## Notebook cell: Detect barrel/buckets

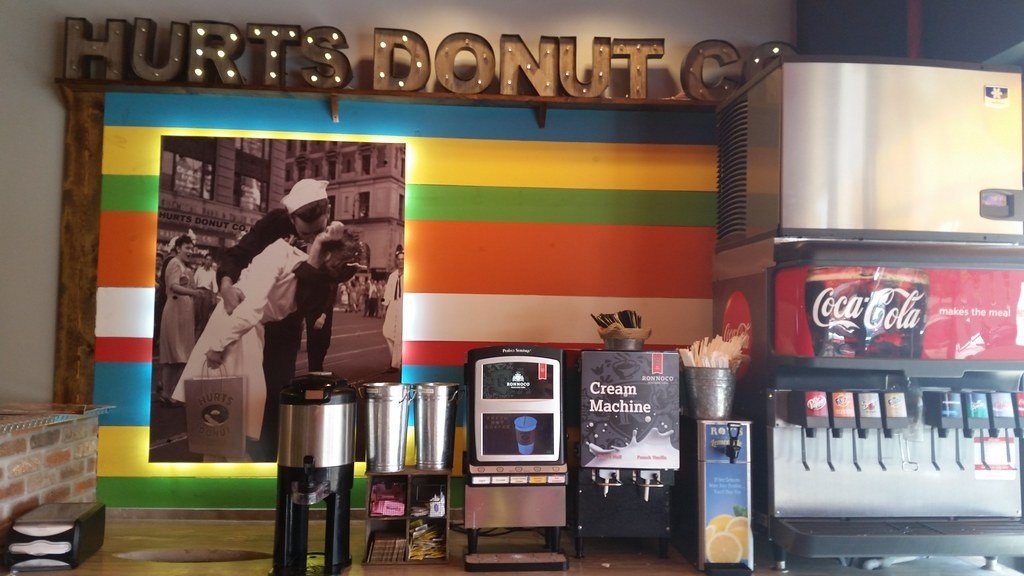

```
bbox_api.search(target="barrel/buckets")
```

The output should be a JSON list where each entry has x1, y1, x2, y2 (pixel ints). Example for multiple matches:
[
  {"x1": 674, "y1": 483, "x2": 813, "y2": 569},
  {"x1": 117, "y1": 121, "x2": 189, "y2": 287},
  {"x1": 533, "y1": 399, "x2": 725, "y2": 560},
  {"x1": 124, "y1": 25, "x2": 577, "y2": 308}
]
[
  {"x1": 680, "y1": 364, "x2": 740, "y2": 419},
  {"x1": 411, "y1": 381, "x2": 460, "y2": 471},
  {"x1": 358, "y1": 381, "x2": 417, "y2": 473}
]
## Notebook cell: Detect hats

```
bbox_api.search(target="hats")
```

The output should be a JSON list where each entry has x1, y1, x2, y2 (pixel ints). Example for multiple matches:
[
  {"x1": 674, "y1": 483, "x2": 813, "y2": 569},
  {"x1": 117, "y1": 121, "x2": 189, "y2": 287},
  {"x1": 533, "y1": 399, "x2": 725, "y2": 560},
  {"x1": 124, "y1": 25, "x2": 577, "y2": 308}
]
[{"x1": 280, "y1": 179, "x2": 329, "y2": 215}]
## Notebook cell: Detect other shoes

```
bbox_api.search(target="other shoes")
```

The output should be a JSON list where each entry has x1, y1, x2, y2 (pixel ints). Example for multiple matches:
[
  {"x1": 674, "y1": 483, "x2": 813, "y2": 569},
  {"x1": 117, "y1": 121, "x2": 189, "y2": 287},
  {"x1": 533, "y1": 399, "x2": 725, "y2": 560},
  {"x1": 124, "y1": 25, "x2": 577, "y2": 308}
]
[
  {"x1": 386, "y1": 367, "x2": 400, "y2": 373},
  {"x1": 159, "y1": 391, "x2": 178, "y2": 405}
]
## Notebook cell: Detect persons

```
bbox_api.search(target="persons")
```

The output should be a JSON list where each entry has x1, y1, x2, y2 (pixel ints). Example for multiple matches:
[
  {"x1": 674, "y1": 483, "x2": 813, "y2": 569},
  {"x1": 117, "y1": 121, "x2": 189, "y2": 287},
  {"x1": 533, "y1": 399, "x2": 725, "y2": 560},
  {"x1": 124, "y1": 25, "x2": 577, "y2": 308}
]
[{"x1": 154, "y1": 177, "x2": 405, "y2": 462}]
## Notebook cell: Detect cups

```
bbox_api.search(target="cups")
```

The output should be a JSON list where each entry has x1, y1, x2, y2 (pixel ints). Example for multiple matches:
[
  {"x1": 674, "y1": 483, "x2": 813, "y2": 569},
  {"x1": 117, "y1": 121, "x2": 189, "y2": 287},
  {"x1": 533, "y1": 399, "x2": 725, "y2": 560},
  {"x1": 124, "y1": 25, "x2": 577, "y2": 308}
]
[
  {"x1": 514, "y1": 417, "x2": 537, "y2": 454},
  {"x1": 804, "y1": 266, "x2": 929, "y2": 359}
]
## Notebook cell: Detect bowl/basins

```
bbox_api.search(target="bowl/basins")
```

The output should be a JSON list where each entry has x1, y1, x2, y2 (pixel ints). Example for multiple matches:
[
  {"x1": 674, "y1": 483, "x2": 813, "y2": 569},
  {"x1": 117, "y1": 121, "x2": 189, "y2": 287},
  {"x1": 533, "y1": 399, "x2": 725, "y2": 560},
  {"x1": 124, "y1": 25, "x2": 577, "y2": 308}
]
[{"x1": 596, "y1": 328, "x2": 652, "y2": 350}]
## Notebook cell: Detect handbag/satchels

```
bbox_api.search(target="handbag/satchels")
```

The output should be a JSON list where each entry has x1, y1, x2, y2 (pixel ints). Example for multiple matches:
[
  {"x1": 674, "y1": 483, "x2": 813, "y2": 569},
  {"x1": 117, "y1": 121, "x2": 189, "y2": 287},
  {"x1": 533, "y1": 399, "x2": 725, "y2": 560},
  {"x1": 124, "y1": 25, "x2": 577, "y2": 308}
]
[{"x1": 185, "y1": 359, "x2": 246, "y2": 457}]
